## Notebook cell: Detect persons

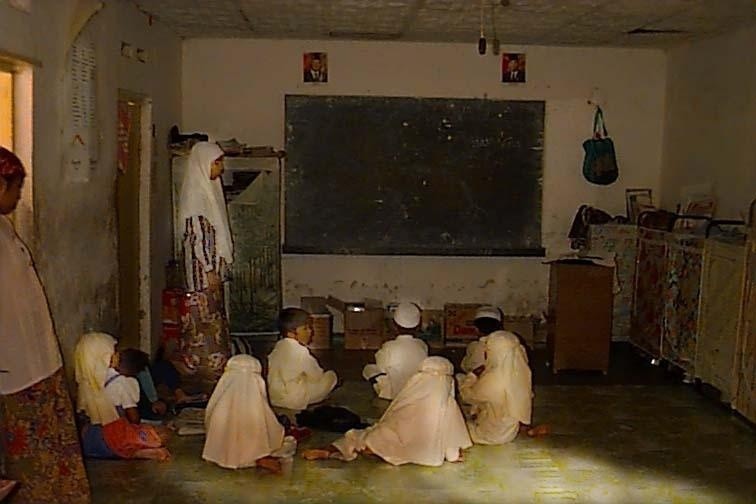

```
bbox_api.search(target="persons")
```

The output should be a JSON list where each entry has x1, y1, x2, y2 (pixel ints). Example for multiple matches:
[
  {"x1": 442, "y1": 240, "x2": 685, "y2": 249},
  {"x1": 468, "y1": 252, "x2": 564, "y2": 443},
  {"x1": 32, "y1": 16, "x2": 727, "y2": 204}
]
[
  {"x1": 502, "y1": 54, "x2": 525, "y2": 82},
  {"x1": 201, "y1": 352, "x2": 300, "y2": 475},
  {"x1": 72, "y1": 330, "x2": 171, "y2": 459},
  {"x1": 267, "y1": 305, "x2": 344, "y2": 413},
  {"x1": 0, "y1": 146, "x2": 95, "y2": 503},
  {"x1": 361, "y1": 302, "x2": 432, "y2": 403},
  {"x1": 304, "y1": 53, "x2": 328, "y2": 82},
  {"x1": 302, "y1": 355, "x2": 475, "y2": 468},
  {"x1": 172, "y1": 141, "x2": 238, "y2": 396},
  {"x1": 116, "y1": 344, "x2": 208, "y2": 423},
  {"x1": 458, "y1": 305, "x2": 503, "y2": 372},
  {"x1": 460, "y1": 329, "x2": 553, "y2": 446}
]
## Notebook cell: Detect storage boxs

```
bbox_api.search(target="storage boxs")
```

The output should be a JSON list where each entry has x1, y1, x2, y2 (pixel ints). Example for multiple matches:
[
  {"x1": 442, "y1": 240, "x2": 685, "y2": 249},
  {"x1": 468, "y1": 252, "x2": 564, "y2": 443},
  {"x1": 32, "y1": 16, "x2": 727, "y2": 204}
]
[{"x1": 299, "y1": 294, "x2": 542, "y2": 350}]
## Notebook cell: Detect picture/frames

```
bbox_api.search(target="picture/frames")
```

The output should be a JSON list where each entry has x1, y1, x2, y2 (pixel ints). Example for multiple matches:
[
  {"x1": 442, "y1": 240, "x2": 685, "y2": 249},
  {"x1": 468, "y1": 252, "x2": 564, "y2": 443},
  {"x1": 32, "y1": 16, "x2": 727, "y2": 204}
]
[
  {"x1": 300, "y1": 49, "x2": 330, "y2": 85},
  {"x1": 499, "y1": 50, "x2": 528, "y2": 85}
]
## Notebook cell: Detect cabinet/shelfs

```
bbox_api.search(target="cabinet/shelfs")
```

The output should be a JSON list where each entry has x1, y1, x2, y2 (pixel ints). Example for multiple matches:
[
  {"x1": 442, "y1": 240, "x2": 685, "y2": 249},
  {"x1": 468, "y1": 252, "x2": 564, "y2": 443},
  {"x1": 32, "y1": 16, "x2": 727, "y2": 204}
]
[{"x1": 169, "y1": 155, "x2": 282, "y2": 340}]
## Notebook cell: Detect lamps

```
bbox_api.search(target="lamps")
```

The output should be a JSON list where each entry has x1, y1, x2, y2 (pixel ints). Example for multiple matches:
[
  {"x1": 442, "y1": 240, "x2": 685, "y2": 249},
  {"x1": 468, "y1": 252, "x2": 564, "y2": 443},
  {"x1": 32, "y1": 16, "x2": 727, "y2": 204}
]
[{"x1": 479, "y1": 5, "x2": 500, "y2": 55}]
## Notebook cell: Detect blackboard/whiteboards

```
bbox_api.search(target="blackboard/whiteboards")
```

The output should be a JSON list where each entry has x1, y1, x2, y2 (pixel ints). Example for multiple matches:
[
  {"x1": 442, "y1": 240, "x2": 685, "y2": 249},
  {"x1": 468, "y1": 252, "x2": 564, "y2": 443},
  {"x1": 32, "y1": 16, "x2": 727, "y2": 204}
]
[{"x1": 281, "y1": 92, "x2": 545, "y2": 257}]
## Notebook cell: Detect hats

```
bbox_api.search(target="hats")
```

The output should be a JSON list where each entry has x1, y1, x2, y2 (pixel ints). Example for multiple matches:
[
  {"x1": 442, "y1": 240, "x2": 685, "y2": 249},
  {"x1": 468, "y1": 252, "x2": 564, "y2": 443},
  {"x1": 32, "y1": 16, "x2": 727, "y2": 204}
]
[
  {"x1": 393, "y1": 303, "x2": 421, "y2": 328},
  {"x1": 1, "y1": 146, "x2": 26, "y2": 174},
  {"x1": 474, "y1": 305, "x2": 501, "y2": 320}
]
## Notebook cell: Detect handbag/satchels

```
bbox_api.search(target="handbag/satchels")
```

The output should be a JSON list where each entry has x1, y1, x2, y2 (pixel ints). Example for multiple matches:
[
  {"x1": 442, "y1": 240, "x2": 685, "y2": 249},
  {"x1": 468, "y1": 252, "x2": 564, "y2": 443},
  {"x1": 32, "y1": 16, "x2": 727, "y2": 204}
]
[
  {"x1": 583, "y1": 136, "x2": 618, "y2": 184},
  {"x1": 568, "y1": 205, "x2": 627, "y2": 248}
]
